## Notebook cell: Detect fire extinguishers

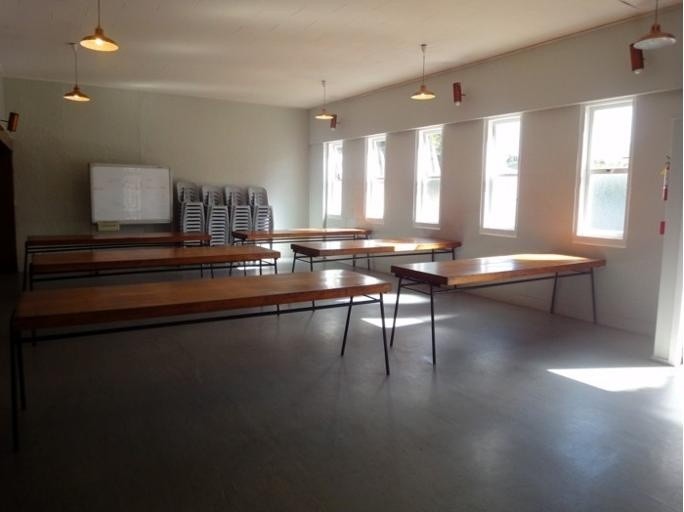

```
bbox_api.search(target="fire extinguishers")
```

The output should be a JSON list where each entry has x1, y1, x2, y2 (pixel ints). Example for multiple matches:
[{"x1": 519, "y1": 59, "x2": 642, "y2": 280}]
[{"x1": 662, "y1": 154, "x2": 671, "y2": 200}]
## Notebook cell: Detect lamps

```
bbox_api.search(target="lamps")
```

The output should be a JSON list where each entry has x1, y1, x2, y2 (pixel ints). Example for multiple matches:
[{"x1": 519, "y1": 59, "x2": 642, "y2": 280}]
[
  {"x1": 631, "y1": 0, "x2": 676, "y2": 50},
  {"x1": 453, "y1": 82, "x2": 466, "y2": 109},
  {"x1": 81, "y1": 0, "x2": 120, "y2": 52},
  {"x1": 1, "y1": 112, "x2": 19, "y2": 132},
  {"x1": 62, "y1": 43, "x2": 92, "y2": 103},
  {"x1": 408, "y1": 44, "x2": 436, "y2": 100},
  {"x1": 330, "y1": 114, "x2": 339, "y2": 130},
  {"x1": 629, "y1": 43, "x2": 644, "y2": 74},
  {"x1": 313, "y1": 79, "x2": 334, "y2": 119}
]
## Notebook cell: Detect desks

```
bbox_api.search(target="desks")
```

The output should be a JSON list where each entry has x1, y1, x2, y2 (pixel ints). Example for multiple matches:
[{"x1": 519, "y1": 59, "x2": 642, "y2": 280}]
[
  {"x1": 389, "y1": 252, "x2": 606, "y2": 365},
  {"x1": 229, "y1": 227, "x2": 371, "y2": 278},
  {"x1": 28, "y1": 246, "x2": 281, "y2": 349},
  {"x1": 21, "y1": 231, "x2": 216, "y2": 291},
  {"x1": 291, "y1": 236, "x2": 463, "y2": 311},
  {"x1": 8, "y1": 270, "x2": 389, "y2": 453}
]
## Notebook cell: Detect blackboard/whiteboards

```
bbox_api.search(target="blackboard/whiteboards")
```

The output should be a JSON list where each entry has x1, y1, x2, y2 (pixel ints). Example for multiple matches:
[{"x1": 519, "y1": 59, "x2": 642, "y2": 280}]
[{"x1": 90, "y1": 163, "x2": 173, "y2": 224}]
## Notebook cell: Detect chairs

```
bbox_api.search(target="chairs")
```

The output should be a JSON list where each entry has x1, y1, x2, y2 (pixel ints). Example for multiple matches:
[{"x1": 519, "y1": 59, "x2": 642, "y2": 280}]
[{"x1": 176, "y1": 181, "x2": 270, "y2": 264}]
